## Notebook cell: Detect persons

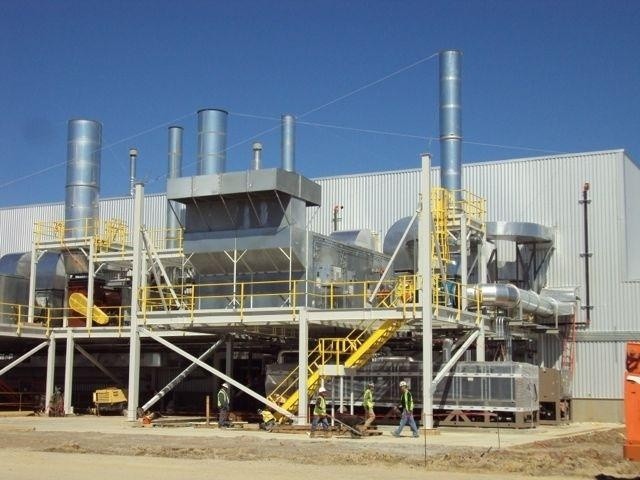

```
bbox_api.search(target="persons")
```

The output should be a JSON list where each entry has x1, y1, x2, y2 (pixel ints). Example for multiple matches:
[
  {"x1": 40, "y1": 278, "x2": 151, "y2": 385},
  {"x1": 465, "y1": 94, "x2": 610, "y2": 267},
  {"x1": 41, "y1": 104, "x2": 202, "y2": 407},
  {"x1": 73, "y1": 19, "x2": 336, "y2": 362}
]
[
  {"x1": 217, "y1": 382, "x2": 230, "y2": 425},
  {"x1": 309, "y1": 386, "x2": 331, "y2": 438},
  {"x1": 257, "y1": 409, "x2": 276, "y2": 432},
  {"x1": 362, "y1": 384, "x2": 375, "y2": 431},
  {"x1": 391, "y1": 381, "x2": 419, "y2": 437}
]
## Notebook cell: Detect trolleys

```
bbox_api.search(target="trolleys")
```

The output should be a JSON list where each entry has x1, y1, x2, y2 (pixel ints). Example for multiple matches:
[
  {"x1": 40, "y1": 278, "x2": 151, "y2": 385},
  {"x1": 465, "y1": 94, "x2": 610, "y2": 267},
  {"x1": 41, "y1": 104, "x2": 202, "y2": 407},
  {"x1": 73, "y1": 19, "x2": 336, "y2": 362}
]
[
  {"x1": 322, "y1": 411, "x2": 365, "y2": 439},
  {"x1": 222, "y1": 419, "x2": 249, "y2": 429}
]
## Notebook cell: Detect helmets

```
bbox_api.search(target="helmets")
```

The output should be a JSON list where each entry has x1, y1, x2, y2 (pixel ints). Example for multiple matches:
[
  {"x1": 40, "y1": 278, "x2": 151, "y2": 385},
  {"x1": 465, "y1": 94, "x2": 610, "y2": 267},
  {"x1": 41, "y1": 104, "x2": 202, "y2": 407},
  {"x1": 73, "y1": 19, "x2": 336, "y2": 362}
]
[
  {"x1": 222, "y1": 383, "x2": 229, "y2": 389},
  {"x1": 399, "y1": 380, "x2": 408, "y2": 387},
  {"x1": 319, "y1": 387, "x2": 327, "y2": 393}
]
